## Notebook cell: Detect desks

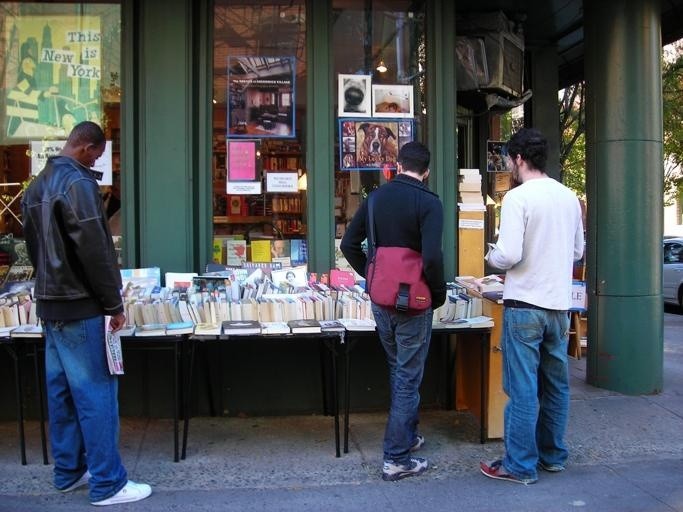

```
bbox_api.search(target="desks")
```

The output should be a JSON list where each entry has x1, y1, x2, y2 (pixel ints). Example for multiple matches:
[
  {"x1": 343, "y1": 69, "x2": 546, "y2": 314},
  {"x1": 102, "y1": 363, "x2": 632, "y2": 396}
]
[
  {"x1": 1, "y1": 315, "x2": 28, "y2": 464},
  {"x1": 28, "y1": 316, "x2": 189, "y2": 466},
  {"x1": 181, "y1": 315, "x2": 346, "y2": 460},
  {"x1": 455, "y1": 276, "x2": 510, "y2": 438},
  {"x1": 342, "y1": 315, "x2": 495, "y2": 454}
]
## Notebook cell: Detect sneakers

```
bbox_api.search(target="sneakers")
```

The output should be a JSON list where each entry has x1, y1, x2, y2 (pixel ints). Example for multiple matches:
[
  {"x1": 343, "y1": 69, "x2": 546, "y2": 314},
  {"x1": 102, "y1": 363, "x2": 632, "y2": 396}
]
[
  {"x1": 91, "y1": 479, "x2": 151, "y2": 506},
  {"x1": 62, "y1": 471, "x2": 92, "y2": 493},
  {"x1": 382, "y1": 434, "x2": 428, "y2": 481},
  {"x1": 480, "y1": 456, "x2": 565, "y2": 484}
]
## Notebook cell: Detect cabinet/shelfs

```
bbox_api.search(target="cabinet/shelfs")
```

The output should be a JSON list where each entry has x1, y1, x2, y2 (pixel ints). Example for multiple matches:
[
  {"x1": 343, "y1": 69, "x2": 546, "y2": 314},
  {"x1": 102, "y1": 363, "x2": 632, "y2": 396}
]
[
  {"x1": 100, "y1": 99, "x2": 345, "y2": 245},
  {"x1": 568, "y1": 246, "x2": 588, "y2": 359},
  {"x1": 459, "y1": 210, "x2": 486, "y2": 278}
]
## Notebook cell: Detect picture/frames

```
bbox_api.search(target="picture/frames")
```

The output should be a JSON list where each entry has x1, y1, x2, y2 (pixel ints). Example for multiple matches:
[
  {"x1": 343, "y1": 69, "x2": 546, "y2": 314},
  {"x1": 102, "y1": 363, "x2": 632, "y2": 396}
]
[
  {"x1": 225, "y1": 54, "x2": 297, "y2": 140},
  {"x1": 372, "y1": 83, "x2": 415, "y2": 117},
  {"x1": 337, "y1": 72, "x2": 373, "y2": 119},
  {"x1": 338, "y1": 116, "x2": 416, "y2": 171}
]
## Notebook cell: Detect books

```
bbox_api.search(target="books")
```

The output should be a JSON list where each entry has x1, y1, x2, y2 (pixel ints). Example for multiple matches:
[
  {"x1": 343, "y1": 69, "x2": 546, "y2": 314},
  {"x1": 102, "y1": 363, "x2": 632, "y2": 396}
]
[
  {"x1": 457, "y1": 168, "x2": 487, "y2": 212},
  {"x1": 431, "y1": 273, "x2": 506, "y2": 328},
  {"x1": 224, "y1": 264, "x2": 377, "y2": 334},
  {"x1": 118, "y1": 268, "x2": 244, "y2": 336},
  {"x1": 483, "y1": 243, "x2": 496, "y2": 262},
  {"x1": 212, "y1": 129, "x2": 308, "y2": 267},
  {"x1": 1, "y1": 265, "x2": 44, "y2": 338}
]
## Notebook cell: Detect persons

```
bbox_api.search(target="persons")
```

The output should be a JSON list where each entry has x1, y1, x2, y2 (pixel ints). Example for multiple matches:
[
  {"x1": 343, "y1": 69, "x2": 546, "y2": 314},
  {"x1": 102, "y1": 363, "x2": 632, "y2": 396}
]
[
  {"x1": 340, "y1": 140, "x2": 447, "y2": 481},
  {"x1": 479, "y1": 128, "x2": 584, "y2": 485},
  {"x1": 21, "y1": 121, "x2": 153, "y2": 506}
]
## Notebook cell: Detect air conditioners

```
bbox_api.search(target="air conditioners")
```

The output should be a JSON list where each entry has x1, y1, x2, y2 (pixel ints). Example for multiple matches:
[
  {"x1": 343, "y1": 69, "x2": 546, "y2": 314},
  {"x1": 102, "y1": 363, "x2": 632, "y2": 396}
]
[{"x1": 471, "y1": 28, "x2": 526, "y2": 99}]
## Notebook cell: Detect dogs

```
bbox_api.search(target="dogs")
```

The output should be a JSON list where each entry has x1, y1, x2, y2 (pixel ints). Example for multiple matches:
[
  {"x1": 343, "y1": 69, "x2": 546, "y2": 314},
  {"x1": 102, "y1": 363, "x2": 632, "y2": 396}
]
[{"x1": 356, "y1": 123, "x2": 398, "y2": 165}]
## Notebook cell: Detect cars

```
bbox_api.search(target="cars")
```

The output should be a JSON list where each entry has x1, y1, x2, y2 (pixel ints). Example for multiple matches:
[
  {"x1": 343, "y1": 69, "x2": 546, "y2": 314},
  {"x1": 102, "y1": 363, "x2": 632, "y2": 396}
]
[{"x1": 663, "y1": 239, "x2": 683, "y2": 310}]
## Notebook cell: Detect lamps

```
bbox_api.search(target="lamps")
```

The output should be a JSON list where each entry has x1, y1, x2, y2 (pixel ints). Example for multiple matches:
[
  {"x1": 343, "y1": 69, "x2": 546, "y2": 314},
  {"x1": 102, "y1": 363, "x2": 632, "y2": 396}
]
[{"x1": 375, "y1": 55, "x2": 388, "y2": 73}]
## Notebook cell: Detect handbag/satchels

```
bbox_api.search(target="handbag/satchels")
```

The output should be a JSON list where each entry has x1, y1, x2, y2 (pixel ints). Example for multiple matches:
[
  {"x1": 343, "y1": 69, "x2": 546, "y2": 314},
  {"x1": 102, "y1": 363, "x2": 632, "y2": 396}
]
[{"x1": 367, "y1": 244, "x2": 433, "y2": 315}]
